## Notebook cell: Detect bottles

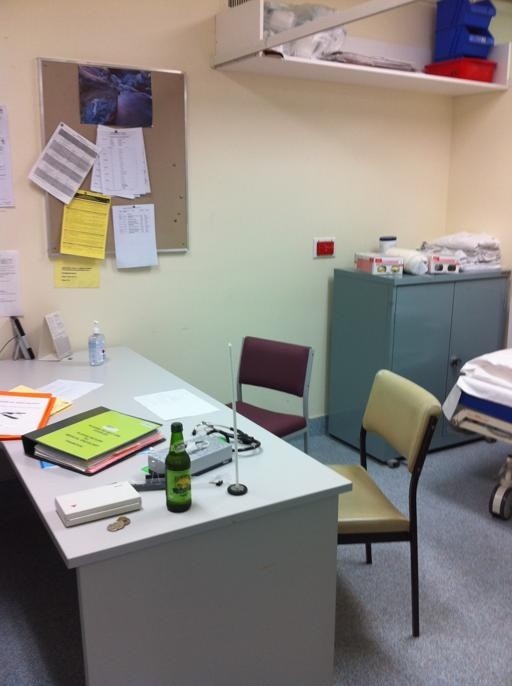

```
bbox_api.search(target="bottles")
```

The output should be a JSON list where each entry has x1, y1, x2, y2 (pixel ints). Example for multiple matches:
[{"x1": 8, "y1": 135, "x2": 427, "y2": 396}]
[
  {"x1": 378, "y1": 235, "x2": 398, "y2": 252},
  {"x1": 86, "y1": 319, "x2": 107, "y2": 367},
  {"x1": 165, "y1": 420, "x2": 192, "y2": 513}
]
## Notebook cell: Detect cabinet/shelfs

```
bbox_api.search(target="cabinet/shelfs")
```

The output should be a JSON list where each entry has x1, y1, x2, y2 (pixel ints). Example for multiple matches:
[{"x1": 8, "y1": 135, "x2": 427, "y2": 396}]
[{"x1": 325, "y1": 266, "x2": 512, "y2": 468}]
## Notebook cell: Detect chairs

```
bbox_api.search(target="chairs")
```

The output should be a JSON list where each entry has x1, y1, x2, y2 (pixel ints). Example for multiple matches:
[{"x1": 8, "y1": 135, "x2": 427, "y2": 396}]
[
  {"x1": 324, "y1": 370, "x2": 442, "y2": 638},
  {"x1": 226, "y1": 336, "x2": 315, "y2": 456}
]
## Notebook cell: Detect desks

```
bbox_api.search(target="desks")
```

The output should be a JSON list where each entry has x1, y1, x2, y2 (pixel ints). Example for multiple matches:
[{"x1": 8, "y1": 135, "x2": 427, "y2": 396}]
[{"x1": 1, "y1": 343, "x2": 354, "y2": 686}]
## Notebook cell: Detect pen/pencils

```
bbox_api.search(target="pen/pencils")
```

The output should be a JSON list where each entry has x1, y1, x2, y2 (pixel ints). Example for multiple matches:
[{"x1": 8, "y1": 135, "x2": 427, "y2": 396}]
[{"x1": 1, "y1": 412, "x2": 26, "y2": 419}]
